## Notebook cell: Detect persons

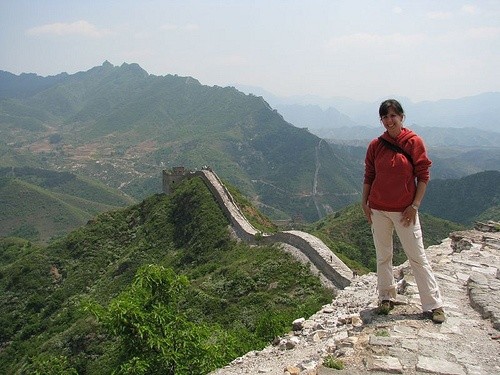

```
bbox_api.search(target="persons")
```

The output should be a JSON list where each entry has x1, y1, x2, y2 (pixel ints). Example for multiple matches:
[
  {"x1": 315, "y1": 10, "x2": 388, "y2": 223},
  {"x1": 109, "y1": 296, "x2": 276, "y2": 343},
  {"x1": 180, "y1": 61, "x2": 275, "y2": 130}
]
[{"x1": 362, "y1": 99, "x2": 445, "y2": 323}]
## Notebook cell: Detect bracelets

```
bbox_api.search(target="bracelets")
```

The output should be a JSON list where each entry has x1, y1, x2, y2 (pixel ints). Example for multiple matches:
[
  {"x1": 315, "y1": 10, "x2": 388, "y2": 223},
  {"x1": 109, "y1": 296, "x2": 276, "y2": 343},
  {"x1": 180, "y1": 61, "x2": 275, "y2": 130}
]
[{"x1": 412, "y1": 204, "x2": 419, "y2": 209}]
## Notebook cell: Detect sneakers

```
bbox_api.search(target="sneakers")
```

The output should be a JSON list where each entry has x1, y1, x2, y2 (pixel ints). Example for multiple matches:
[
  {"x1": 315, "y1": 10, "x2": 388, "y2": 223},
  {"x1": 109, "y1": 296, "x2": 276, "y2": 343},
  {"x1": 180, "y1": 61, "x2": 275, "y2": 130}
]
[
  {"x1": 378, "y1": 300, "x2": 394, "y2": 315},
  {"x1": 432, "y1": 307, "x2": 445, "y2": 322}
]
